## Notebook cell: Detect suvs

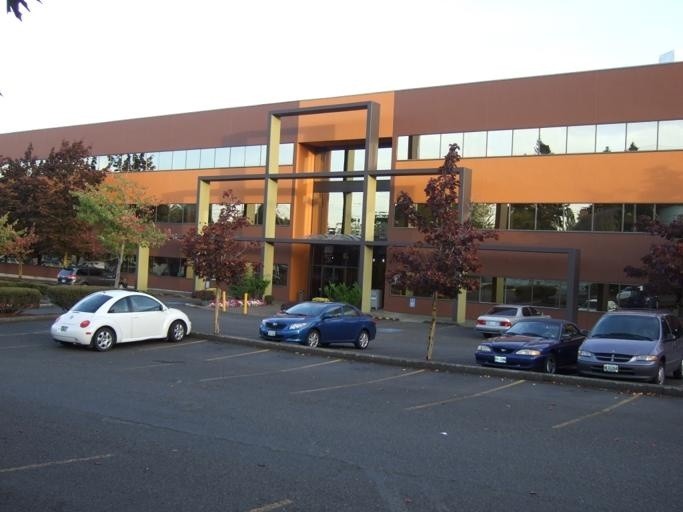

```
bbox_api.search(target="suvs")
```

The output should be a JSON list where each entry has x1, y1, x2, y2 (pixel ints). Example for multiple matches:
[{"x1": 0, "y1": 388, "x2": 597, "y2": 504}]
[{"x1": 58, "y1": 261, "x2": 128, "y2": 291}]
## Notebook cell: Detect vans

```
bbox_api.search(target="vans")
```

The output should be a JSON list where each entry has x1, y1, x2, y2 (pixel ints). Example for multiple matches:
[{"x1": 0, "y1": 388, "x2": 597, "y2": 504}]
[{"x1": 577, "y1": 307, "x2": 682, "y2": 385}]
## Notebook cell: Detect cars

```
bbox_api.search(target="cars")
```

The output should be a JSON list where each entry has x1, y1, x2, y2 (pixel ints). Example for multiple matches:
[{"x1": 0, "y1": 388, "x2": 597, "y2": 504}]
[
  {"x1": 49, "y1": 289, "x2": 193, "y2": 351},
  {"x1": 477, "y1": 304, "x2": 553, "y2": 342},
  {"x1": 477, "y1": 318, "x2": 588, "y2": 377},
  {"x1": 258, "y1": 298, "x2": 377, "y2": 352},
  {"x1": 579, "y1": 286, "x2": 660, "y2": 312}
]
